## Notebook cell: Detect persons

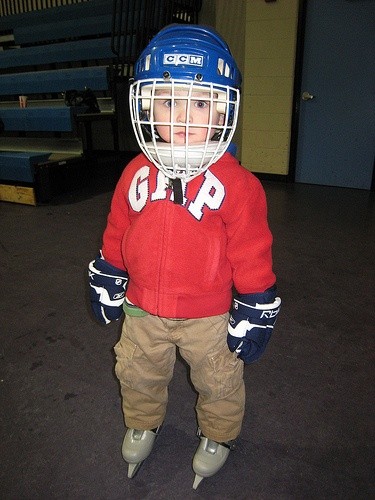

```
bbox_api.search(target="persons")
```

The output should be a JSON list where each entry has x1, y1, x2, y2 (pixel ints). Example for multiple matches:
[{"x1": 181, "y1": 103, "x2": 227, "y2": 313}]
[{"x1": 89, "y1": 24, "x2": 280, "y2": 478}]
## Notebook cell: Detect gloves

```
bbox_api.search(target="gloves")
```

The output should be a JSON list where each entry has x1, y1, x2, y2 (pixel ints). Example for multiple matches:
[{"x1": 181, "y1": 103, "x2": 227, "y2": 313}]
[
  {"x1": 227, "y1": 296, "x2": 281, "y2": 365},
  {"x1": 87, "y1": 260, "x2": 129, "y2": 326}
]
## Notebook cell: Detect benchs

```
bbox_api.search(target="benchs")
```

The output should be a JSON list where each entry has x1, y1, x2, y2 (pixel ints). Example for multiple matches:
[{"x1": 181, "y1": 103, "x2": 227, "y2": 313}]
[{"x1": 0, "y1": 0, "x2": 202, "y2": 208}]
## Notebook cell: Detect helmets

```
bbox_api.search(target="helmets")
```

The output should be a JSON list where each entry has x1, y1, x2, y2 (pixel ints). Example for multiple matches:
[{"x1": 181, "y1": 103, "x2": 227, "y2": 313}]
[{"x1": 129, "y1": 21, "x2": 240, "y2": 167}]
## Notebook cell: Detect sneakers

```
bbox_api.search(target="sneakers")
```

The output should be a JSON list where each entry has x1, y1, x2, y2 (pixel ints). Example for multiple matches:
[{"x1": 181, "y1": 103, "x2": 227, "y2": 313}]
[
  {"x1": 192, "y1": 427, "x2": 237, "y2": 490},
  {"x1": 122, "y1": 426, "x2": 160, "y2": 478}
]
